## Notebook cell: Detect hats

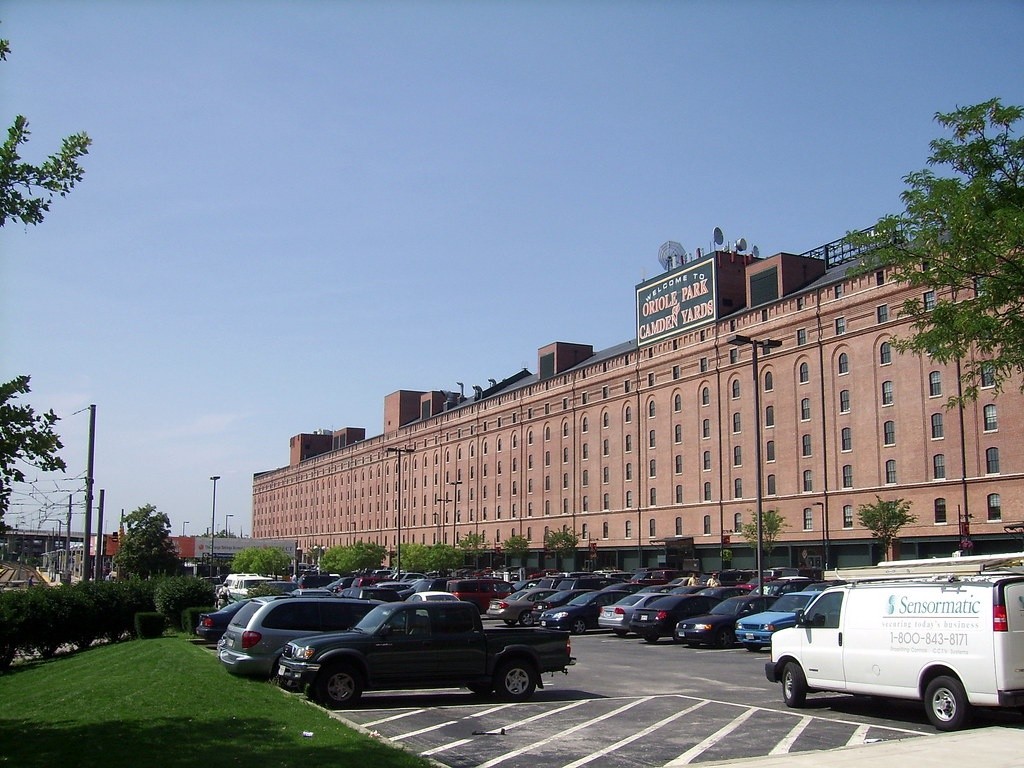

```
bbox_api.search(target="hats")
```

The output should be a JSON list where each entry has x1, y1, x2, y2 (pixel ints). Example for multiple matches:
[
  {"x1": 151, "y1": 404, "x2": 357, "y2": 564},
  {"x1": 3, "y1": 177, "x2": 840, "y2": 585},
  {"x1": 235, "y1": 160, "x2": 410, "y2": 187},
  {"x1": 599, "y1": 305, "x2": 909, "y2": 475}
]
[
  {"x1": 223, "y1": 582, "x2": 228, "y2": 585},
  {"x1": 712, "y1": 572, "x2": 717, "y2": 576}
]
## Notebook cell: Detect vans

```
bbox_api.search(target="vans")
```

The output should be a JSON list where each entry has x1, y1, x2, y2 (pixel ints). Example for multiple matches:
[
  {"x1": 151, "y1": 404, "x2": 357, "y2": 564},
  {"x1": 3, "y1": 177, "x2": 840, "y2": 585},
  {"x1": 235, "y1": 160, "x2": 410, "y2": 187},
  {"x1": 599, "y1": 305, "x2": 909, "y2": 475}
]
[{"x1": 762, "y1": 570, "x2": 1023, "y2": 732}]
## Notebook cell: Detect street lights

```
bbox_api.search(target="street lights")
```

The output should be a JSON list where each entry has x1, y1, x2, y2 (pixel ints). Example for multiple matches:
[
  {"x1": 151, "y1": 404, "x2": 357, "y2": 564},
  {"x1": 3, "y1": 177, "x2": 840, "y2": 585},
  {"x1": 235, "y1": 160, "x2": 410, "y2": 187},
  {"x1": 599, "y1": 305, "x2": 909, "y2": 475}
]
[
  {"x1": 813, "y1": 501, "x2": 826, "y2": 570},
  {"x1": 448, "y1": 481, "x2": 463, "y2": 549},
  {"x1": 385, "y1": 447, "x2": 416, "y2": 582},
  {"x1": 726, "y1": 334, "x2": 782, "y2": 597},
  {"x1": 314, "y1": 545, "x2": 326, "y2": 575},
  {"x1": 209, "y1": 475, "x2": 221, "y2": 592},
  {"x1": 225, "y1": 514, "x2": 234, "y2": 538},
  {"x1": 437, "y1": 498, "x2": 452, "y2": 546},
  {"x1": 182, "y1": 521, "x2": 190, "y2": 537}
]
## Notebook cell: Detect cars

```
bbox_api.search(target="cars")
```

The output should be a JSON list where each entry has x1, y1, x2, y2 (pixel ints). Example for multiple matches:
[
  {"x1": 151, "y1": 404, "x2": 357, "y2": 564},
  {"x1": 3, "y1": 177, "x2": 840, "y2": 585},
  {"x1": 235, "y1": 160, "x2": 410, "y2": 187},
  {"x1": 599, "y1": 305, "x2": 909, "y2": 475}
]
[{"x1": 192, "y1": 568, "x2": 846, "y2": 691}]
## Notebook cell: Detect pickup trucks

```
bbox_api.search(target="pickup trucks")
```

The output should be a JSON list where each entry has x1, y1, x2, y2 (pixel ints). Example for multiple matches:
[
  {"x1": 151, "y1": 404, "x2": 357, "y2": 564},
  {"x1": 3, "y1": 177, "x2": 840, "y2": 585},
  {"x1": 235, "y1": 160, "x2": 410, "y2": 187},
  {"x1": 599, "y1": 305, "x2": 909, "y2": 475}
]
[{"x1": 277, "y1": 598, "x2": 577, "y2": 710}]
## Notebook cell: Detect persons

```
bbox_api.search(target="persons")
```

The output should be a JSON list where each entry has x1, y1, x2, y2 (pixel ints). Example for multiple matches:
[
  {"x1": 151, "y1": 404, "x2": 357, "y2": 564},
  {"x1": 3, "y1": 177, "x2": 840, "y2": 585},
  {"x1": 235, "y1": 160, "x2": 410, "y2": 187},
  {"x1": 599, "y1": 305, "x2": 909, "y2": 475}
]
[
  {"x1": 292, "y1": 573, "x2": 298, "y2": 584},
  {"x1": 688, "y1": 573, "x2": 701, "y2": 586},
  {"x1": 298, "y1": 574, "x2": 306, "y2": 589},
  {"x1": 707, "y1": 573, "x2": 721, "y2": 588},
  {"x1": 28, "y1": 575, "x2": 35, "y2": 587},
  {"x1": 218, "y1": 582, "x2": 231, "y2": 611}
]
